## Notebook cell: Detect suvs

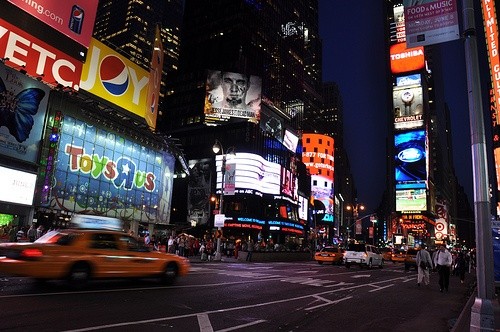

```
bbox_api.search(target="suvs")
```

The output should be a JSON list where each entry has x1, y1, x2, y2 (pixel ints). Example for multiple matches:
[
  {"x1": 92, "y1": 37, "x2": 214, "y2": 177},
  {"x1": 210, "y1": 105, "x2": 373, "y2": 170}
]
[
  {"x1": 343, "y1": 244, "x2": 384, "y2": 270},
  {"x1": 379, "y1": 247, "x2": 392, "y2": 261},
  {"x1": 403, "y1": 247, "x2": 424, "y2": 272}
]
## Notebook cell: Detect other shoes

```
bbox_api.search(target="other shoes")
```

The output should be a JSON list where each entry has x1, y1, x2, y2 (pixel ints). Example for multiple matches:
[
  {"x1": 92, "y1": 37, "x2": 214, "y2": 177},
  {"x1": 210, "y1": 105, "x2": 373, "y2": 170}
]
[
  {"x1": 440, "y1": 288, "x2": 443, "y2": 293},
  {"x1": 445, "y1": 288, "x2": 448, "y2": 292}
]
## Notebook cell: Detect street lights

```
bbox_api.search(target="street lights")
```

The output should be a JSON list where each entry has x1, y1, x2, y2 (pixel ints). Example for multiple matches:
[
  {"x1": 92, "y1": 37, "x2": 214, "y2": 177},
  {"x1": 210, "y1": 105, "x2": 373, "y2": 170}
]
[
  {"x1": 347, "y1": 202, "x2": 364, "y2": 239},
  {"x1": 213, "y1": 138, "x2": 225, "y2": 262}
]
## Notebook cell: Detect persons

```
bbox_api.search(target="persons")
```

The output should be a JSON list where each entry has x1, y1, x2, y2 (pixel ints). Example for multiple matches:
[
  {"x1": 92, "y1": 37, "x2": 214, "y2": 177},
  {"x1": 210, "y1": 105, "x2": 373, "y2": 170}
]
[
  {"x1": 401, "y1": 243, "x2": 477, "y2": 293},
  {"x1": 211, "y1": 71, "x2": 253, "y2": 111},
  {"x1": 130, "y1": 231, "x2": 325, "y2": 262},
  {"x1": 0, "y1": 223, "x2": 58, "y2": 243}
]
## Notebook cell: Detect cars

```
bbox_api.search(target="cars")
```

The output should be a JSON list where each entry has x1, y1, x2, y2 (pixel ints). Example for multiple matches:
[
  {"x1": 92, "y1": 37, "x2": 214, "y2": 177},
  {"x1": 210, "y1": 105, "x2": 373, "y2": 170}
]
[
  {"x1": 314, "y1": 245, "x2": 345, "y2": 264},
  {"x1": 0, "y1": 216, "x2": 191, "y2": 286},
  {"x1": 391, "y1": 250, "x2": 407, "y2": 264}
]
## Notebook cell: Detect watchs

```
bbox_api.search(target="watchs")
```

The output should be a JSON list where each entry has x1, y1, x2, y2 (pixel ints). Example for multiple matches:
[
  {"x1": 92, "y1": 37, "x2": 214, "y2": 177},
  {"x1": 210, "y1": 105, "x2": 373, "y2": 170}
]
[{"x1": 402, "y1": 87, "x2": 414, "y2": 118}]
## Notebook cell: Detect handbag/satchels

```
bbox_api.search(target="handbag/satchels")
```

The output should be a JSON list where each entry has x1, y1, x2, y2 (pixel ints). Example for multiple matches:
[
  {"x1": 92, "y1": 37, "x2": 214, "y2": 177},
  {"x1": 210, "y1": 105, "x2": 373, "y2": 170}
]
[{"x1": 420, "y1": 260, "x2": 428, "y2": 269}]
